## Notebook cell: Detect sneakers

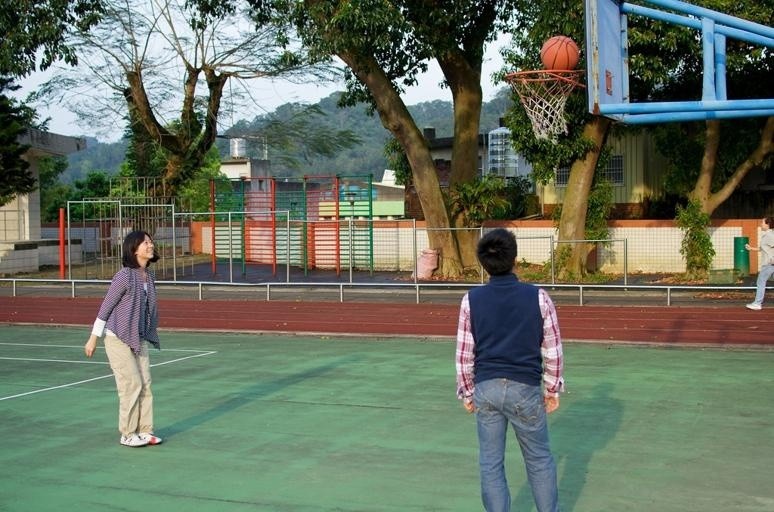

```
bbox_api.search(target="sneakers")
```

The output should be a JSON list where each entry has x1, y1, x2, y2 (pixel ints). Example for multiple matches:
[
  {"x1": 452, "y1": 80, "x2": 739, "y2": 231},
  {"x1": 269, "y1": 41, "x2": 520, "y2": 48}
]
[
  {"x1": 120, "y1": 432, "x2": 149, "y2": 446},
  {"x1": 746, "y1": 301, "x2": 762, "y2": 311},
  {"x1": 137, "y1": 432, "x2": 162, "y2": 444}
]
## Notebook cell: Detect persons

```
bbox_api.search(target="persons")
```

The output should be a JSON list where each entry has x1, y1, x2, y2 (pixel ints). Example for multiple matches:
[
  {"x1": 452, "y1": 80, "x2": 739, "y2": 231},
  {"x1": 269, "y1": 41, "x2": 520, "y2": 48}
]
[
  {"x1": 452, "y1": 227, "x2": 565, "y2": 512},
  {"x1": 744, "y1": 214, "x2": 774, "y2": 312},
  {"x1": 83, "y1": 230, "x2": 164, "y2": 448}
]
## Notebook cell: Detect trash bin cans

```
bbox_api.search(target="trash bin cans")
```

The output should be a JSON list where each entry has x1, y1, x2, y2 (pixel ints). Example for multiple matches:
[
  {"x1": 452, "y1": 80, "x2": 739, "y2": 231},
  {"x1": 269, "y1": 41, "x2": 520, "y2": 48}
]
[{"x1": 734, "y1": 237, "x2": 749, "y2": 277}]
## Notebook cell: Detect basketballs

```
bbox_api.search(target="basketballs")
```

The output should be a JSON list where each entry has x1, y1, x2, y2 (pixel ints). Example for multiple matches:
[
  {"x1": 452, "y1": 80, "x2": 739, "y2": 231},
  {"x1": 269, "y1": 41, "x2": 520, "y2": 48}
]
[{"x1": 541, "y1": 36, "x2": 579, "y2": 72}]
